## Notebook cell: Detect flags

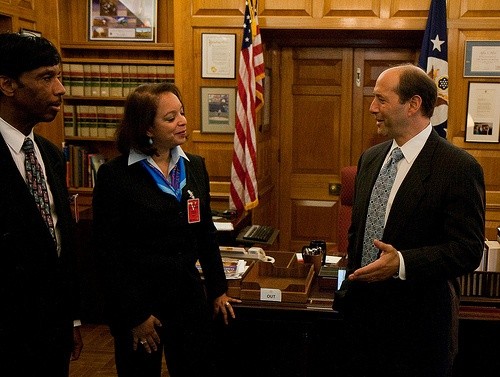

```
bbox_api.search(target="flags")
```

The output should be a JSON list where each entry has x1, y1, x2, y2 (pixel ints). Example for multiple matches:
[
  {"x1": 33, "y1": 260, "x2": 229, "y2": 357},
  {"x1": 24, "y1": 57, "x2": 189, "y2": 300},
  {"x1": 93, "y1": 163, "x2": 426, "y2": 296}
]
[
  {"x1": 417, "y1": 0, "x2": 450, "y2": 140},
  {"x1": 228, "y1": 1, "x2": 266, "y2": 228}
]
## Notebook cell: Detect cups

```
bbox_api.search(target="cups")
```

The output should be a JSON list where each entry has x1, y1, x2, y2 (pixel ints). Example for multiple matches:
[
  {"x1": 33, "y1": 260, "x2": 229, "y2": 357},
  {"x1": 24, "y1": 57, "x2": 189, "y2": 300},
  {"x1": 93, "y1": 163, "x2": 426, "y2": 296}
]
[
  {"x1": 301, "y1": 240, "x2": 327, "y2": 266},
  {"x1": 303, "y1": 252, "x2": 322, "y2": 278}
]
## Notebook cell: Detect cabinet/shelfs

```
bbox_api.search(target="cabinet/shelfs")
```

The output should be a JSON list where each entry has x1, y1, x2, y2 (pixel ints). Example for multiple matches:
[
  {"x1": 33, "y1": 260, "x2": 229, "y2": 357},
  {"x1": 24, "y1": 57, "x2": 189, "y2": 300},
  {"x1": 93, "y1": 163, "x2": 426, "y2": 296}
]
[
  {"x1": 219, "y1": 251, "x2": 316, "y2": 302},
  {"x1": 61, "y1": 57, "x2": 175, "y2": 219}
]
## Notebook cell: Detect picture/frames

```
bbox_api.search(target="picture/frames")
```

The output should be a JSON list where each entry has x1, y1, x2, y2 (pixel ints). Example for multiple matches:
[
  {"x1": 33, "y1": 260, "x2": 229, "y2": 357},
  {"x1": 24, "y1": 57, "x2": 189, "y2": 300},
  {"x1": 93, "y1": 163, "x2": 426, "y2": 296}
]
[
  {"x1": 85, "y1": 0, "x2": 158, "y2": 43},
  {"x1": 199, "y1": 85, "x2": 238, "y2": 135},
  {"x1": 20, "y1": 27, "x2": 42, "y2": 37}
]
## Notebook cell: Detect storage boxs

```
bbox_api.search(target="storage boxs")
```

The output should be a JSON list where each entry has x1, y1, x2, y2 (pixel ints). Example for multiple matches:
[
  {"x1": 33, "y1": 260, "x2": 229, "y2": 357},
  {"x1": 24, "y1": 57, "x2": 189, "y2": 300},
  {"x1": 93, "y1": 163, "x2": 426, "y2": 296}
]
[
  {"x1": 205, "y1": 254, "x2": 500, "y2": 377},
  {"x1": 456, "y1": 239, "x2": 500, "y2": 307}
]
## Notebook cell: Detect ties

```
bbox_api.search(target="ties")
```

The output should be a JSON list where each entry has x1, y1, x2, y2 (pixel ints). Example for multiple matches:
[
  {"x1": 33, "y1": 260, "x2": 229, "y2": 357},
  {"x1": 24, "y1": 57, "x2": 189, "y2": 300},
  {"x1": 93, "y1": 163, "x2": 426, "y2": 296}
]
[
  {"x1": 20, "y1": 137, "x2": 58, "y2": 253},
  {"x1": 360, "y1": 148, "x2": 405, "y2": 267}
]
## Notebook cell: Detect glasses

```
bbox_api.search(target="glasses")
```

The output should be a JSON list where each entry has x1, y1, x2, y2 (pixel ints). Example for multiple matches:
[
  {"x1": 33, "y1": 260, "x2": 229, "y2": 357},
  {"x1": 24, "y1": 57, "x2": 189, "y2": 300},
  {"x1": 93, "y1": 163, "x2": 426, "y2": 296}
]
[{"x1": 18, "y1": 29, "x2": 43, "y2": 47}]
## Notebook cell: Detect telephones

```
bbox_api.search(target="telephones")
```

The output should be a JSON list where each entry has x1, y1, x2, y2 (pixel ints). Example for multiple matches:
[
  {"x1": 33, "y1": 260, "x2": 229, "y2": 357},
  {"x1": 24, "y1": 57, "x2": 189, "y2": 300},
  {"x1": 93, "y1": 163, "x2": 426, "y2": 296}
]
[{"x1": 219, "y1": 245, "x2": 268, "y2": 262}]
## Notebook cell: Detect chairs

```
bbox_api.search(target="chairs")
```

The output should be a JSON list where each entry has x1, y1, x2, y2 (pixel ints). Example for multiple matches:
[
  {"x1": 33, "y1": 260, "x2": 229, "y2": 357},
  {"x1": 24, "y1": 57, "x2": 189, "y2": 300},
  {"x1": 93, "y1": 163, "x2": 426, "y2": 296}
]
[{"x1": 327, "y1": 165, "x2": 358, "y2": 257}]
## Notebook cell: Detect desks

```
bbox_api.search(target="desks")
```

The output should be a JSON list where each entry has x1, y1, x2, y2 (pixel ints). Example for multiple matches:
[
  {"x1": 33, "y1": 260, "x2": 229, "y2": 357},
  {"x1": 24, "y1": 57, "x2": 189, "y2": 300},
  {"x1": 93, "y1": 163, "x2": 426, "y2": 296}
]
[{"x1": 212, "y1": 207, "x2": 280, "y2": 249}]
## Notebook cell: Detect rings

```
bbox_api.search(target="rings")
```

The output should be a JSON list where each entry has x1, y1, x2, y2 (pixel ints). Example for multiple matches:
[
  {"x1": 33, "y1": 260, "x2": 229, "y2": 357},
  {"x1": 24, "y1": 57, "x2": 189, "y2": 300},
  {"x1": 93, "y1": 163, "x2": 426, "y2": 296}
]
[
  {"x1": 225, "y1": 302, "x2": 229, "y2": 306},
  {"x1": 140, "y1": 341, "x2": 147, "y2": 344}
]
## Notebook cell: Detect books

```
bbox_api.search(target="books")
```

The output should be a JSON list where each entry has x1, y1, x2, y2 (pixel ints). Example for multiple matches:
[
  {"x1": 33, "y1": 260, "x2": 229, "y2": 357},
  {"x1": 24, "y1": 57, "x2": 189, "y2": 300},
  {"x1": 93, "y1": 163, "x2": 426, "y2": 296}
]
[
  {"x1": 61, "y1": 141, "x2": 107, "y2": 190},
  {"x1": 60, "y1": 63, "x2": 176, "y2": 99},
  {"x1": 456, "y1": 227, "x2": 500, "y2": 297},
  {"x1": 62, "y1": 105, "x2": 126, "y2": 139}
]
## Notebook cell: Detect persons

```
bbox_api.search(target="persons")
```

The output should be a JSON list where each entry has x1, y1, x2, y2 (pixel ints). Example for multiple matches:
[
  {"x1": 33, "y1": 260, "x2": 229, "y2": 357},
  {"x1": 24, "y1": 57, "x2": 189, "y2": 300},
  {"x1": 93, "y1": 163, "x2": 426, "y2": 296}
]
[
  {"x1": 0, "y1": 31, "x2": 84, "y2": 377},
  {"x1": 332, "y1": 64, "x2": 487, "y2": 377},
  {"x1": 91, "y1": 83, "x2": 244, "y2": 377}
]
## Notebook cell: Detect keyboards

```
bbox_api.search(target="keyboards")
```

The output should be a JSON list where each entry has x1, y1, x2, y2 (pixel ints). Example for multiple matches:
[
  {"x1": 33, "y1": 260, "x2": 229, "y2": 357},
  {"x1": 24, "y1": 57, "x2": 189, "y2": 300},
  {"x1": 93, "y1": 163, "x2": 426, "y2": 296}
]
[{"x1": 242, "y1": 224, "x2": 273, "y2": 241}]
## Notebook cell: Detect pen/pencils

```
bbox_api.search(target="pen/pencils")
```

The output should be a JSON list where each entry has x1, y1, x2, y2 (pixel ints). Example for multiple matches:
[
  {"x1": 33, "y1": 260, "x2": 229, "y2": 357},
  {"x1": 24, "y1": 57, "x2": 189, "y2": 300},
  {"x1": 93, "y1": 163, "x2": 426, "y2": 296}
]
[{"x1": 305, "y1": 247, "x2": 323, "y2": 255}]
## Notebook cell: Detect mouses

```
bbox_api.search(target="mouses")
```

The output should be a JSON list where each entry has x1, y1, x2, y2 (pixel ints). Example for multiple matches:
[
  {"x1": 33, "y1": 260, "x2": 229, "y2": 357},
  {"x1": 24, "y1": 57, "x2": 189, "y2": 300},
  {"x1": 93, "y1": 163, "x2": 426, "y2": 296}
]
[{"x1": 223, "y1": 210, "x2": 237, "y2": 215}]
